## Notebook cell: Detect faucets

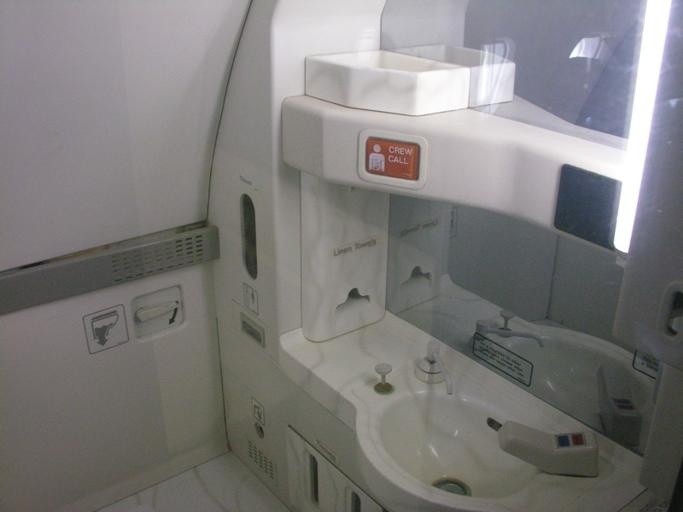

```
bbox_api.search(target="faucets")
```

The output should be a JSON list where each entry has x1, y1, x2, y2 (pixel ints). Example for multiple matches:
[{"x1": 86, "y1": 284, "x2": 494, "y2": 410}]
[
  {"x1": 476, "y1": 309, "x2": 545, "y2": 349},
  {"x1": 415, "y1": 341, "x2": 453, "y2": 394}
]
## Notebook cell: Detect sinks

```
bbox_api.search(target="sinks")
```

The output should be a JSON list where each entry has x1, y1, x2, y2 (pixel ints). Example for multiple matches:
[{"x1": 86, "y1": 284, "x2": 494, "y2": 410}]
[
  {"x1": 378, "y1": 392, "x2": 541, "y2": 500},
  {"x1": 507, "y1": 338, "x2": 654, "y2": 457}
]
